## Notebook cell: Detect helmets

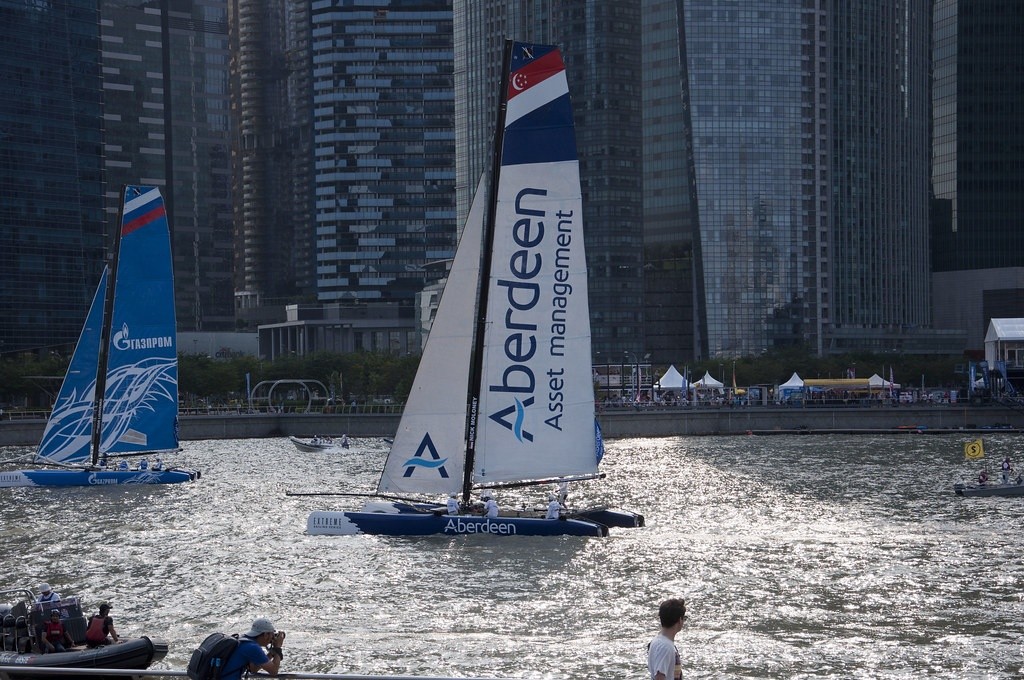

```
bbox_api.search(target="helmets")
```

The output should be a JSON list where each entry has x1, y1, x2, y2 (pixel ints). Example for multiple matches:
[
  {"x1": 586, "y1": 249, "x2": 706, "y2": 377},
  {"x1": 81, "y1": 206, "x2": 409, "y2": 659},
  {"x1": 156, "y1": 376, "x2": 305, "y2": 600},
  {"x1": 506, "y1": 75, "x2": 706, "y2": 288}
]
[
  {"x1": 548, "y1": 495, "x2": 555, "y2": 501},
  {"x1": 38, "y1": 582, "x2": 51, "y2": 592},
  {"x1": 450, "y1": 492, "x2": 456, "y2": 497},
  {"x1": 50, "y1": 609, "x2": 60, "y2": 616}
]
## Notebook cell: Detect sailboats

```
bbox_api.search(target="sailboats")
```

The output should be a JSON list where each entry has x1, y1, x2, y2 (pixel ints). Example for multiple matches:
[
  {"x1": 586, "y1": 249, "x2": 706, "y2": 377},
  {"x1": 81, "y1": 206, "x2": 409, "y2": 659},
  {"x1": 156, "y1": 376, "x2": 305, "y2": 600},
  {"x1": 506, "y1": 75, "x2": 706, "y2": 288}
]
[
  {"x1": 0, "y1": 183, "x2": 201, "y2": 487},
  {"x1": 307, "y1": 38, "x2": 645, "y2": 537}
]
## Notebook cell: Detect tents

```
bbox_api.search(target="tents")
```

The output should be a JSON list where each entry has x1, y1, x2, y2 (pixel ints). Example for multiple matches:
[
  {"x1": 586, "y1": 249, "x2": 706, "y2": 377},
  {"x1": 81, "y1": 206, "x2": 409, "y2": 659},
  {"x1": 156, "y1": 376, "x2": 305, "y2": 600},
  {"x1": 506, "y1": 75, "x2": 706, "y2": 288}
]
[
  {"x1": 869, "y1": 374, "x2": 900, "y2": 388},
  {"x1": 777, "y1": 373, "x2": 813, "y2": 391},
  {"x1": 651, "y1": 364, "x2": 724, "y2": 391}
]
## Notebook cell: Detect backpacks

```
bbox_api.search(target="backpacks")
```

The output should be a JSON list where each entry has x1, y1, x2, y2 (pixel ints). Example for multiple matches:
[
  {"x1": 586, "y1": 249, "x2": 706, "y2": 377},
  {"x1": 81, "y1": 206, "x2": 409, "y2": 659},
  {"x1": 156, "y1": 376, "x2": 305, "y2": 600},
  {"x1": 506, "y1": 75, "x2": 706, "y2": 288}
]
[{"x1": 187, "y1": 632, "x2": 254, "y2": 680}]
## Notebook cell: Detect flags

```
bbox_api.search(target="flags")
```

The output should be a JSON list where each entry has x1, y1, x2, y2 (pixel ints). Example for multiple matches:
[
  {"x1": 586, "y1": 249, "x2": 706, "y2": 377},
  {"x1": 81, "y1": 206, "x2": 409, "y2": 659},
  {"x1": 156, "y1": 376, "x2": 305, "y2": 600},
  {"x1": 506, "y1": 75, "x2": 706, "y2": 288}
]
[{"x1": 964, "y1": 438, "x2": 984, "y2": 459}]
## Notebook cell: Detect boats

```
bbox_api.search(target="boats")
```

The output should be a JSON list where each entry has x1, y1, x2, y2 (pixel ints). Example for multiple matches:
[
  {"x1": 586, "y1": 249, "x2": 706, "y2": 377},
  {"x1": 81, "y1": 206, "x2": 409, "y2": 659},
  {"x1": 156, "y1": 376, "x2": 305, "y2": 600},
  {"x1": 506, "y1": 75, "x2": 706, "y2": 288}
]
[
  {"x1": 384, "y1": 438, "x2": 394, "y2": 448},
  {"x1": 289, "y1": 434, "x2": 349, "y2": 453},
  {"x1": 953, "y1": 437, "x2": 1024, "y2": 497},
  {"x1": 0, "y1": 589, "x2": 169, "y2": 680}
]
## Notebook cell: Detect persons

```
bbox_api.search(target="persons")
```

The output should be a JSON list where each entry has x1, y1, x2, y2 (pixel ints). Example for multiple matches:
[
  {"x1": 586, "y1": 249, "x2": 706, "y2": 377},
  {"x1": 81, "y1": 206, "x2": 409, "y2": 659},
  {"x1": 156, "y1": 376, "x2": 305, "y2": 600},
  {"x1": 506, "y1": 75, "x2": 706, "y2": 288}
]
[
  {"x1": 187, "y1": 619, "x2": 286, "y2": 680},
  {"x1": 557, "y1": 476, "x2": 568, "y2": 509},
  {"x1": 351, "y1": 400, "x2": 356, "y2": 414},
  {"x1": 37, "y1": 583, "x2": 61, "y2": 619},
  {"x1": 100, "y1": 451, "x2": 109, "y2": 466},
  {"x1": 599, "y1": 390, "x2": 951, "y2": 409},
  {"x1": 447, "y1": 492, "x2": 460, "y2": 516},
  {"x1": 152, "y1": 457, "x2": 161, "y2": 471},
  {"x1": 545, "y1": 496, "x2": 565, "y2": 519},
  {"x1": 1002, "y1": 456, "x2": 1013, "y2": 482},
  {"x1": 85, "y1": 604, "x2": 123, "y2": 648},
  {"x1": 979, "y1": 470, "x2": 987, "y2": 488},
  {"x1": 483, "y1": 495, "x2": 498, "y2": 517},
  {"x1": 311, "y1": 435, "x2": 333, "y2": 445},
  {"x1": 139, "y1": 457, "x2": 148, "y2": 471},
  {"x1": 647, "y1": 599, "x2": 688, "y2": 680},
  {"x1": 41, "y1": 609, "x2": 78, "y2": 653}
]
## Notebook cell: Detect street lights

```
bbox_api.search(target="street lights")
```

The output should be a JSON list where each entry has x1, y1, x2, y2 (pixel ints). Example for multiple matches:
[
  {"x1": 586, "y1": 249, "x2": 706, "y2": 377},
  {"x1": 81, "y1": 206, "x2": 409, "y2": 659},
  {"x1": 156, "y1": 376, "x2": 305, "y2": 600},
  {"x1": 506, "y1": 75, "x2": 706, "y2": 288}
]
[
  {"x1": 624, "y1": 351, "x2": 654, "y2": 403},
  {"x1": 594, "y1": 352, "x2": 629, "y2": 402}
]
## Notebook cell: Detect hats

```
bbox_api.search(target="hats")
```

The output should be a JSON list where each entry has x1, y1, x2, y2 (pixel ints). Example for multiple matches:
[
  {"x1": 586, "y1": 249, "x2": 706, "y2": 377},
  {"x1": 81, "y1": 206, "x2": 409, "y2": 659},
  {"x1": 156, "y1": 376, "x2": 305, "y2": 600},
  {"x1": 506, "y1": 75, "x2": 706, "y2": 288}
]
[
  {"x1": 243, "y1": 618, "x2": 275, "y2": 637},
  {"x1": 100, "y1": 604, "x2": 114, "y2": 610}
]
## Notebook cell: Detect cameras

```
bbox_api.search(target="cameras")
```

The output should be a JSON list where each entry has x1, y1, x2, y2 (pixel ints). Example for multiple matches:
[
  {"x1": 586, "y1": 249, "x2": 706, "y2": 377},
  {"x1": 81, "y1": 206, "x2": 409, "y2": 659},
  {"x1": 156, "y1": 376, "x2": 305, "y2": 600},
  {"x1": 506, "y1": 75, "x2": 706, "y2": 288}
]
[{"x1": 272, "y1": 632, "x2": 286, "y2": 644}]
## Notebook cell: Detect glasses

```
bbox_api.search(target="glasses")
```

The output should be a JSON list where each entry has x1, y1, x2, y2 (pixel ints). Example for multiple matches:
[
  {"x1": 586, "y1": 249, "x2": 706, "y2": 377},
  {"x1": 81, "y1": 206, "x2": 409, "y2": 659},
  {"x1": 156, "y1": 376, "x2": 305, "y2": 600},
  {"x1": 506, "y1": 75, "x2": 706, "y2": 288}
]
[{"x1": 682, "y1": 616, "x2": 688, "y2": 621}]
